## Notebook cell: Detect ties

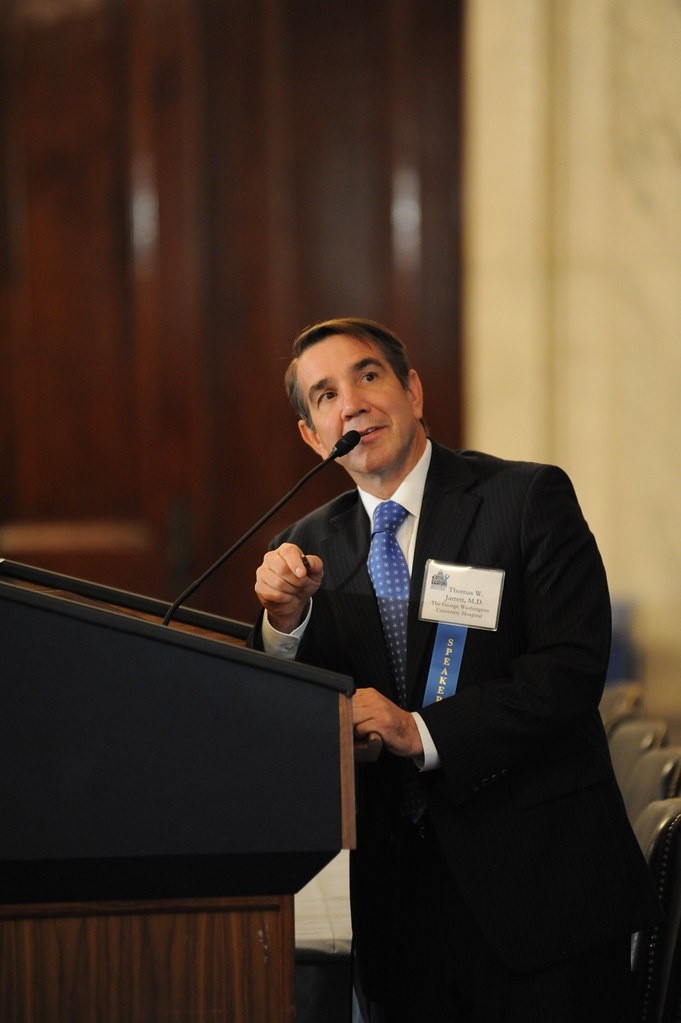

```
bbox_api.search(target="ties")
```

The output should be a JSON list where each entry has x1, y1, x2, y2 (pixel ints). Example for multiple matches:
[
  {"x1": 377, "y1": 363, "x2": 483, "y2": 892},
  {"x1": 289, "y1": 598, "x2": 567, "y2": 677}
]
[{"x1": 365, "y1": 501, "x2": 429, "y2": 825}]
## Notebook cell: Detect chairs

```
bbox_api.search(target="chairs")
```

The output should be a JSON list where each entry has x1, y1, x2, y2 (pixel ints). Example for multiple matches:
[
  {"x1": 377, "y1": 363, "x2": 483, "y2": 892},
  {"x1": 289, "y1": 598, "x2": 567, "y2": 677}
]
[{"x1": 598, "y1": 683, "x2": 681, "y2": 1023}]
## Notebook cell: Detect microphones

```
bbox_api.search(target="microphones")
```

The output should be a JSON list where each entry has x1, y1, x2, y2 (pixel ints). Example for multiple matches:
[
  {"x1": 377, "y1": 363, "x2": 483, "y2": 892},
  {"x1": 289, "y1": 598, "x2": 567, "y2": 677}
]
[{"x1": 163, "y1": 430, "x2": 361, "y2": 625}]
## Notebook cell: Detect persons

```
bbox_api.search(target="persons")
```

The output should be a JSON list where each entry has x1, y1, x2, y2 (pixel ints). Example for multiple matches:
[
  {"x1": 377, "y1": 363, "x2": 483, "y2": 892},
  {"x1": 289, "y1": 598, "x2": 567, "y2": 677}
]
[{"x1": 246, "y1": 318, "x2": 659, "y2": 1023}]
